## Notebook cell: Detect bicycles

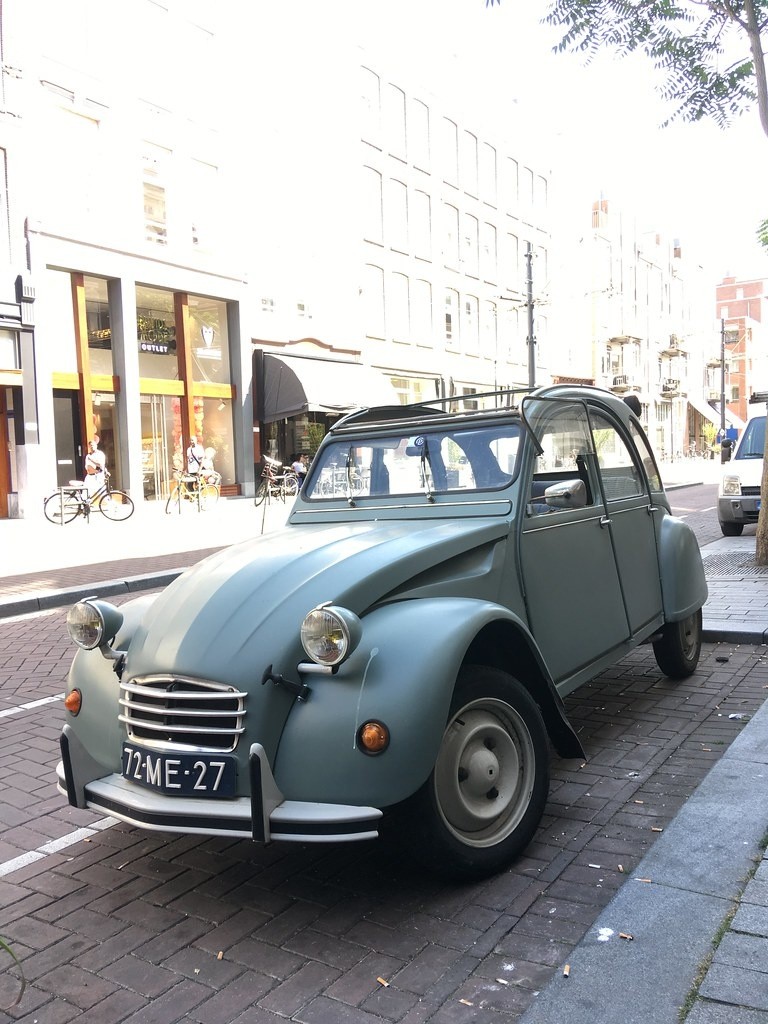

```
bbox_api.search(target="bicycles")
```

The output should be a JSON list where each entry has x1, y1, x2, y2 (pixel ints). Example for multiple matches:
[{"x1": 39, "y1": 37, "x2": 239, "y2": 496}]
[
  {"x1": 42, "y1": 467, "x2": 135, "y2": 524},
  {"x1": 319, "y1": 471, "x2": 364, "y2": 496},
  {"x1": 254, "y1": 455, "x2": 299, "y2": 506},
  {"x1": 165, "y1": 467, "x2": 218, "y2": 514}
]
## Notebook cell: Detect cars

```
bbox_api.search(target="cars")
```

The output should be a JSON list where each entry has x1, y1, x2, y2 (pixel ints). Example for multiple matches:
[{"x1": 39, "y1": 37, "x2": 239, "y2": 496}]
[{"x1": 54, "y1": 384, "x2": 708, "y2": 878}]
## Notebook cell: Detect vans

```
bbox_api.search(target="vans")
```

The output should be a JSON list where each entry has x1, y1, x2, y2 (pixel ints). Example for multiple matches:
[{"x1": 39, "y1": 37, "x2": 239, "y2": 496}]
[{"x1": 716, "y1": 416, "x2": 768, "y2": 536}]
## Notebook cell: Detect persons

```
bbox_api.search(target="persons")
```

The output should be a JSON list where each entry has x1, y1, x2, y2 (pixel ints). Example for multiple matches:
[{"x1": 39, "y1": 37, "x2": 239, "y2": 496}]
[
  {"x1": 181, "y1": 436, "x2": 204, "y2": 501},
  {"x1": 202, "y1": 448, "x2": 222, "y2": 485},
  {"x1": 336, "y1": 455, "x2": 348, "y2": 467},
  {"x1": 291, "y1": 453, "x2": 307, "y2": 493},
  {"x1": 81, "y1": 441, "x2": 115, "y2": 511}
]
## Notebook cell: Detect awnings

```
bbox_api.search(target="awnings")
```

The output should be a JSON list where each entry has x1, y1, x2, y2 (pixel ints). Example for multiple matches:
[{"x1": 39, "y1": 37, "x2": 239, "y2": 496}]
[
  {"x1": 263, "y1": 353, "x2": 400, "y2": 424},
  {"x1": 689, "y1": 400, "x2": 745, "y2": 429}
]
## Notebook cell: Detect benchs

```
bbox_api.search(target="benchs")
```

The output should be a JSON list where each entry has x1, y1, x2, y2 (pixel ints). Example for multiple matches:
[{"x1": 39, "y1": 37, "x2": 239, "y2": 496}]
[{"x1": 529, "y1": 475, "x2": 638, "y2": 509}]
